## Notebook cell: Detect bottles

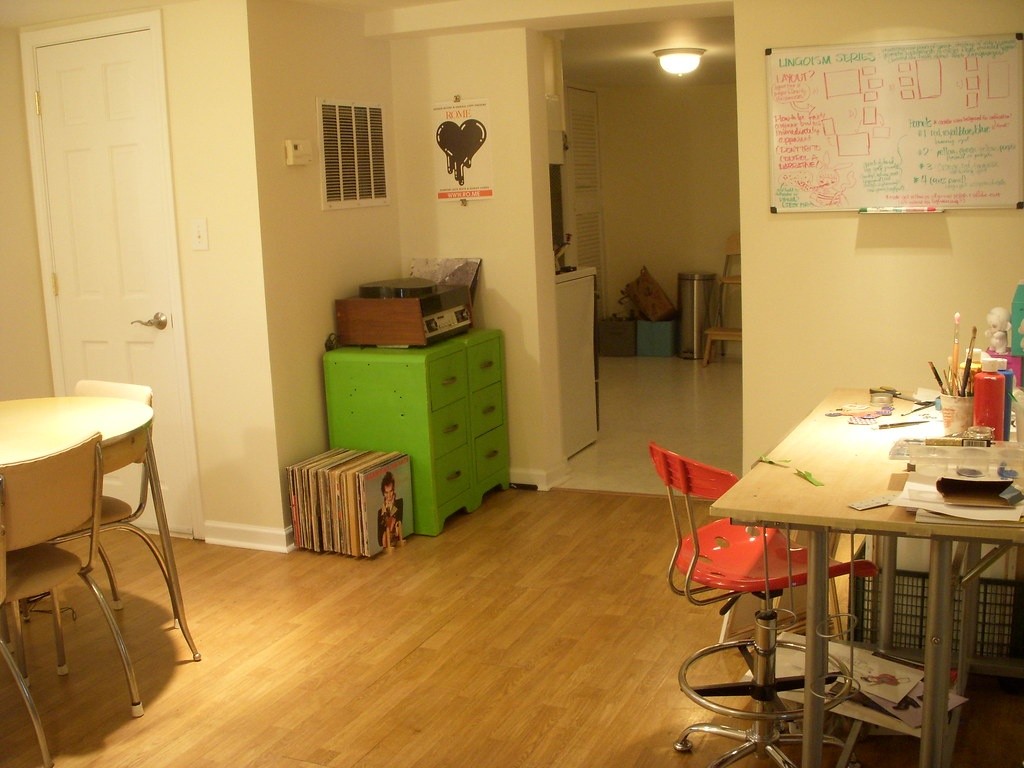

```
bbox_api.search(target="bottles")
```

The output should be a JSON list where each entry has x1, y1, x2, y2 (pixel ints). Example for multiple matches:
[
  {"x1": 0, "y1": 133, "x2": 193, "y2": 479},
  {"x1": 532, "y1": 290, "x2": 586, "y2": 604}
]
[
  {"x1": 997, "y1": 359, "x2": 1014, "y2": 441},
  {"x1": 960, "y1": 349, "x2": 981, "y2": 393},
  {"x1": 973, "y1": 358, "x2": 1004, "y2": 441}
]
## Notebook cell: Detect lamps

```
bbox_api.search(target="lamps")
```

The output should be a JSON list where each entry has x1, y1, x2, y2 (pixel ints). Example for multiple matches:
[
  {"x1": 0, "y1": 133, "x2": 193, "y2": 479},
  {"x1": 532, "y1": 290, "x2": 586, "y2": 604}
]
[{"x1": 653, "y1": 48, "x2": 707, "y2": 76}]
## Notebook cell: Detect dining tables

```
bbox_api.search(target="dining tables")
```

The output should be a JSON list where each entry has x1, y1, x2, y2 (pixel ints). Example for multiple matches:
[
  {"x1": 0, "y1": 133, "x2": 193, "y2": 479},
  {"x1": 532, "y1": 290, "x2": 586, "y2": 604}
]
[{"x1": 0, "y1": 398, "x2": 201, "y2": 667}]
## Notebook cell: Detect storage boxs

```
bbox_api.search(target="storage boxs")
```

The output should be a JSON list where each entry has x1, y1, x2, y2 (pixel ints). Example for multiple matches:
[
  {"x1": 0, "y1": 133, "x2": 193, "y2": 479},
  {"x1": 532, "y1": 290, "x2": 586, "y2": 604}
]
[
  {"x1": 636, "y1": 313, "x2": 681, "y2": 356},
  {"x1": 848, "y1": 537, "x2": 1024, "y2": 694},
  {"x1": 600, "y1": 317, "x2": 637, "y2": 357}
]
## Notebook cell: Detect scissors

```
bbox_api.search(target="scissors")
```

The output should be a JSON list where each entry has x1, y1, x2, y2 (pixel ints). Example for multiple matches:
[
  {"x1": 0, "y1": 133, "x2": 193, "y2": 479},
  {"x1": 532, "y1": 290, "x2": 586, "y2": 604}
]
[{"x1": 870, "y1": 384, "x2": 921, "y2": 404}]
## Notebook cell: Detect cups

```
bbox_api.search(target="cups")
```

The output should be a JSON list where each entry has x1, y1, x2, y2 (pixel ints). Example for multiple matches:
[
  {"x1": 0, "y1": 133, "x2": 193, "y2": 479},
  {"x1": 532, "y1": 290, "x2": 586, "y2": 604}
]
[
  {"x1": 941, "y1": 389, "x2": 974, "y2": 436},
  {"x1": 957, "y1": 449, "x2": 988, "y2": 477}
]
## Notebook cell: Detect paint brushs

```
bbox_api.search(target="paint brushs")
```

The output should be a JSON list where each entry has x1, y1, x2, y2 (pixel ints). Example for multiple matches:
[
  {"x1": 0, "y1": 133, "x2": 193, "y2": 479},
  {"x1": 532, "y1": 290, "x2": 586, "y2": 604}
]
[{"x1": 927, "y1": 311, "x2": 977, "y2": 397}]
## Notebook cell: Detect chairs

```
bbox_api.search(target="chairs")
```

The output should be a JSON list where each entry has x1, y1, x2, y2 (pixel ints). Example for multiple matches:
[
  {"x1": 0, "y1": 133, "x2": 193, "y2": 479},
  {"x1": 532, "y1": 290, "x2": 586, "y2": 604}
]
[
  {"x1": 647, "y1": 440, "x2": 880, "y2": 768},
  {"x1": 0, "y1": 432, "x2": 144, "y2": 768},
  {"x1": 703, "y1": 233, "x2": 742, "y2": 366},
  {"x1": 19, "y1": 379, "x2": 179, "y2": 676}
]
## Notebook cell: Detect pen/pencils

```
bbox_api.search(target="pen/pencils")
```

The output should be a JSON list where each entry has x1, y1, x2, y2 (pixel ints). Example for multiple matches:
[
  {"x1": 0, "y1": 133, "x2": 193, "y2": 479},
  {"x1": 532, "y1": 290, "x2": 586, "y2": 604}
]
[
  {"x1": 871, "y1": 420, "x2": 930, "y2": 430},
  {"x1": 901, "y1": 402, "x2": 935, "y2": 416}
]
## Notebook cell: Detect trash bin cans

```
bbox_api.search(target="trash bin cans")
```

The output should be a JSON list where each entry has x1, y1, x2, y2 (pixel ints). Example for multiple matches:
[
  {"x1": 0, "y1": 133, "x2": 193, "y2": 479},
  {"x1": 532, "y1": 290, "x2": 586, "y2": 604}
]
[{"x1": 678, "y1": 272, "x2": 716, "y2": 359}]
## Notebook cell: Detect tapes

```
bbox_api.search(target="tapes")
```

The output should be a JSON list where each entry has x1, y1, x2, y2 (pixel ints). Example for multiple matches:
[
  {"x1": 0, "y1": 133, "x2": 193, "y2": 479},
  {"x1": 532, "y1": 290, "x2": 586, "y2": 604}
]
[{"x1": 871, "y1": 393, "x2": 893, "y2": 404}]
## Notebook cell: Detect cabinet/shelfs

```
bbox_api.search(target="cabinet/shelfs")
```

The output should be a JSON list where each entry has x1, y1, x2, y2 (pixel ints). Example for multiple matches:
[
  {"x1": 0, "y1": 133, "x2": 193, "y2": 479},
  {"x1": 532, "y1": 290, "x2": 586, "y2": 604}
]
[{"x1": 323, "y1": 329, "x2": 511, "y2": 536}]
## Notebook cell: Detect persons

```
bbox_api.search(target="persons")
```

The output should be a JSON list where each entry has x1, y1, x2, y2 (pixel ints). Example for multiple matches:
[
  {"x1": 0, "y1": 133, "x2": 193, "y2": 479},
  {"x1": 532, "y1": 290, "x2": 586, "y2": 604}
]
[{"x1": 377, "y1": 472, "x2": 404, "y2": 547}]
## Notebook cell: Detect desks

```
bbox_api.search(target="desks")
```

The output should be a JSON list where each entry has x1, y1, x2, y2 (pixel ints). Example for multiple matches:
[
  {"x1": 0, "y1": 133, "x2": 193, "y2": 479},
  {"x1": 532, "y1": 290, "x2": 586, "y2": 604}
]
[{"x1": 709, "y1": 389, "x2": 1023, "y2": 768}]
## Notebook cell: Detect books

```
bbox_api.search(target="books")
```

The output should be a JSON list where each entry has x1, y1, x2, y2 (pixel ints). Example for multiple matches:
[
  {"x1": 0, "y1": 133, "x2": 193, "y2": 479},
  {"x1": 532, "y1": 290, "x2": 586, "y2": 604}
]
[
  {"x1": 287, "y1": 447, "x2": 415, "y2": 558},
  {"x1": 915, "y1": 508, "x2": 1024, "y2": 528}
]
